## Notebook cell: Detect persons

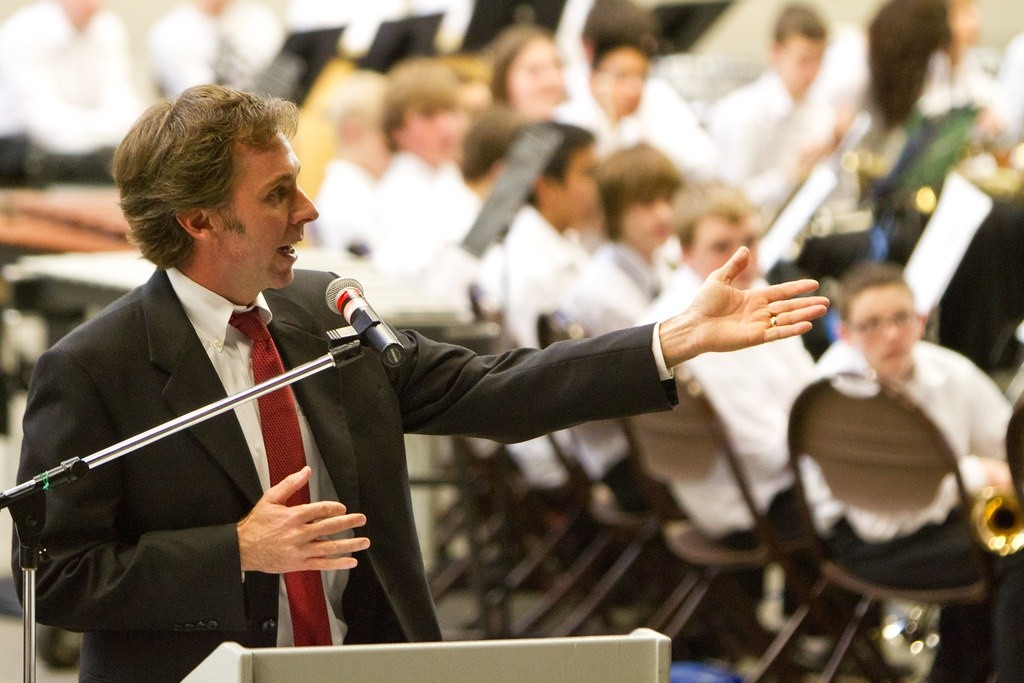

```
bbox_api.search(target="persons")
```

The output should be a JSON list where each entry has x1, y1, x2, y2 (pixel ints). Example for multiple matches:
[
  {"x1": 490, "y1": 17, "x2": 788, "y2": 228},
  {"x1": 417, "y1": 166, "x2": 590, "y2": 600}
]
[
  {"x1": 299, "y1": 0, "x2": 1021, "y2": 679},
  {"x1": 16, "y1": 86, "x2": 830, "y2": 681}
]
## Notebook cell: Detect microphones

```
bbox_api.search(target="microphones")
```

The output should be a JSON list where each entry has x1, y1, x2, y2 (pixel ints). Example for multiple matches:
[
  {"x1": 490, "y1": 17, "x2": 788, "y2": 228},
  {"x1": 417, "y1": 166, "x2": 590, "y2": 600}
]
[{"x1": 326, "y1": 278, "x2": 406, "y2": 367}]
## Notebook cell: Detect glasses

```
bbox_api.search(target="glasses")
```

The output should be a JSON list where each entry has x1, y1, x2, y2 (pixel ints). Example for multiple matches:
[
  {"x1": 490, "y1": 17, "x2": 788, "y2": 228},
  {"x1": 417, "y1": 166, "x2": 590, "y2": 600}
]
[{"x1": 850, "y1": 309, "x2": 914, "y2": 331}]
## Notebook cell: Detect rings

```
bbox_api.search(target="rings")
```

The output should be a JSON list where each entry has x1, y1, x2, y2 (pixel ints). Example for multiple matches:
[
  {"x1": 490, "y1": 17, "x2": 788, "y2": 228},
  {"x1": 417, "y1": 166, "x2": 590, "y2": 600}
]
[{"x1": 771, "y1": 316, "x2": 778, "y2": 325}]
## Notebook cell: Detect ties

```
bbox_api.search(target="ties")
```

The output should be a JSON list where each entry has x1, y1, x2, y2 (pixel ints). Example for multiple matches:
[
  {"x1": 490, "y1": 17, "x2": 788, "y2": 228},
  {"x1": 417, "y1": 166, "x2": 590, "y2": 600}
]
[{"x1": 227, "y1": 306, "x2": 333, "y2": 646}]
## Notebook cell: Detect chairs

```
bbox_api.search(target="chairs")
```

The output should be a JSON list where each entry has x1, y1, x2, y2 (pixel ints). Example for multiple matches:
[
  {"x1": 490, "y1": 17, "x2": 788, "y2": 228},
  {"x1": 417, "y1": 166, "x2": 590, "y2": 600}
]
[
  {"x1": 613, "y1": 361, "x2": 875, "y2": 683},
  {"x1": 454, "y1": 282, "x2": 676, "y2": 638},
  {"x1": 787, "y1": 369, "x2": 1003, "y2": 683},
  {"x1": 536, "y1": 313, "x2": 763, "y2": 658}
]
